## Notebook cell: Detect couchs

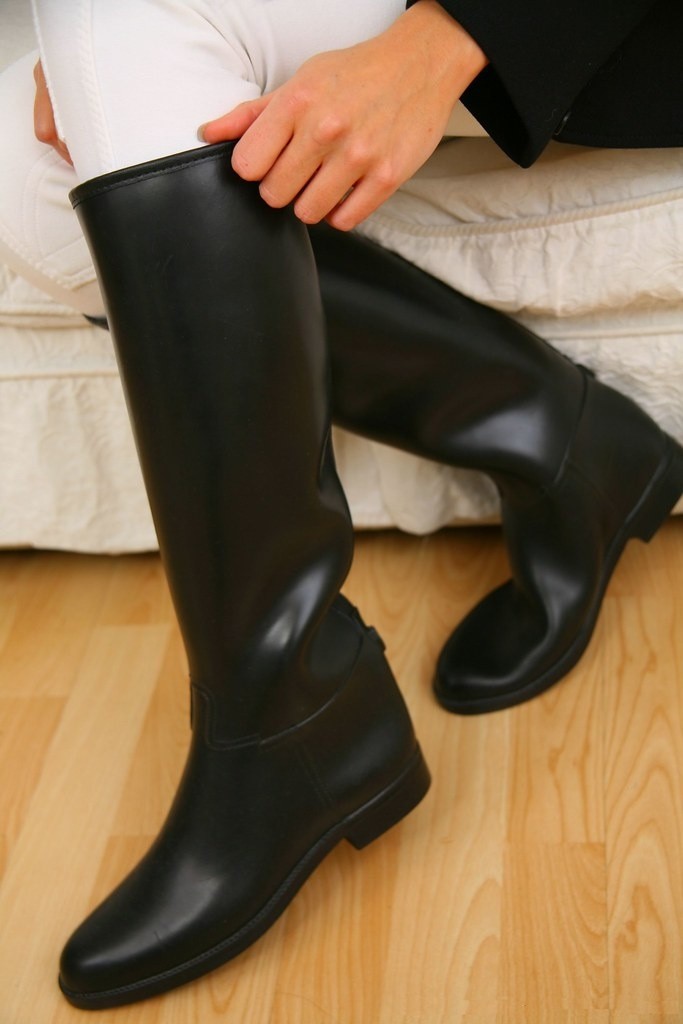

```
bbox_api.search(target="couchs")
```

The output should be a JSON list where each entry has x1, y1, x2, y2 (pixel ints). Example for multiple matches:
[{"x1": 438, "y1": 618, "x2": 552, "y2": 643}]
[{"x1": 1, "y1": 0, "x2": 682, "y2": 555}]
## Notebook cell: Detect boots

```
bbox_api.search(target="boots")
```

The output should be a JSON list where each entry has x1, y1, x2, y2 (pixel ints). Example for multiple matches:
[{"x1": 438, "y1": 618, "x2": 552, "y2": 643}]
[
  {"x1": 82, "y1": 221, "x2": 683, "y2": 715},
  {"x1": 58, "y1": 140, "x2": 431, "y2": 1011}
]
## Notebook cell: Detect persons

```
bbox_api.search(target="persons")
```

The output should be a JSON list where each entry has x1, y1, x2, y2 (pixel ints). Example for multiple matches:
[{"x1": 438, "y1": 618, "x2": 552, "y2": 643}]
[{"x1": 26, "y1": 0, "x2": 683, "y2": 1010}]
